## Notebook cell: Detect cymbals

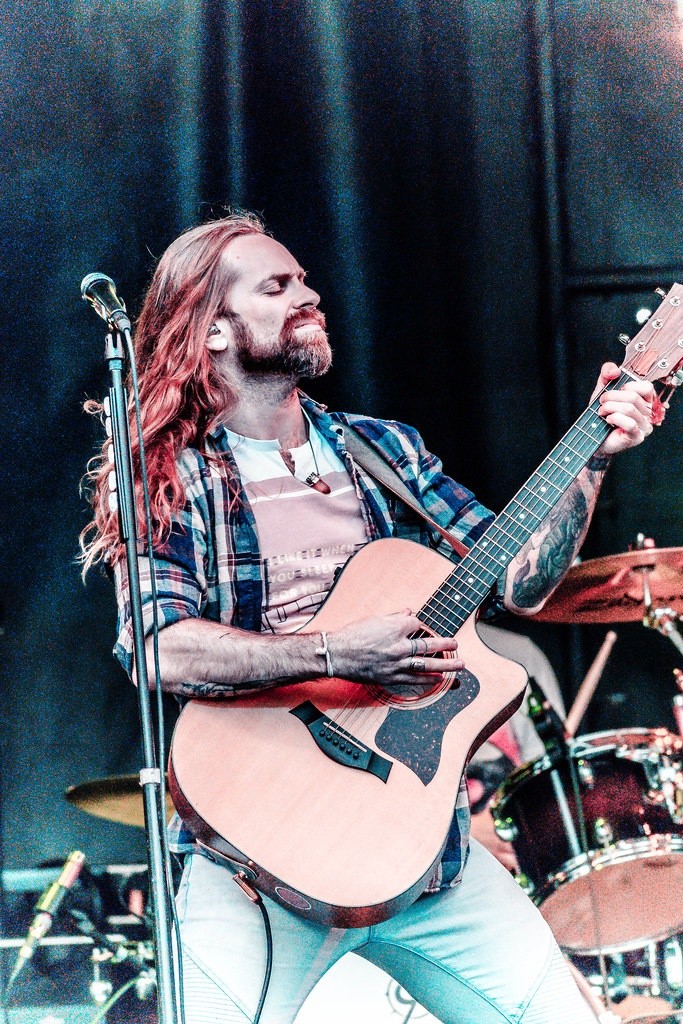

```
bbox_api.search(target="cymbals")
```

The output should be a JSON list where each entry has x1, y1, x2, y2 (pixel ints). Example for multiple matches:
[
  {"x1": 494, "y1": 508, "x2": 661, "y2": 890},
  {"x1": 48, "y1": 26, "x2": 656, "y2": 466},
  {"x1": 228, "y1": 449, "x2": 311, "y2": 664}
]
[
  {"x1": 65, "y1": 771, "x2": 179, "y2": 835},
  {"x1": 530, "y1": 549, "x2": 681, "y2": 626}
]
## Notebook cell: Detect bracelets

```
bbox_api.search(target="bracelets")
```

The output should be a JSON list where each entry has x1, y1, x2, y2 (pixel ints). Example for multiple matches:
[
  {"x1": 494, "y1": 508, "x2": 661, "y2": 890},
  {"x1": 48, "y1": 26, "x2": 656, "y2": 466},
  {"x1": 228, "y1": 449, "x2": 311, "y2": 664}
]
[
  {"x1": 315, "y1": 632, "x2": 333, "y2": 676},
  {"x1": 586, "y1": 455, "x2": 612, "y2": 472}
]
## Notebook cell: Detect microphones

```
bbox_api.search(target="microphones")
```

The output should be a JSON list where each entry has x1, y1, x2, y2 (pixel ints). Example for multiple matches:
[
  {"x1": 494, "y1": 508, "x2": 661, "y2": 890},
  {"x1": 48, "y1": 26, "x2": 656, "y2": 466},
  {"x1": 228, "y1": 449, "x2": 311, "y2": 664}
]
[
  {"x1": 529, "y1": 677, "x2": 566, "y2": 736},
  {"x1": 81, "y1": 272, "x2": 130, "y2": 332},
  {"x1": 16, "y1": 852, "x2": 86, "y2": 969}
]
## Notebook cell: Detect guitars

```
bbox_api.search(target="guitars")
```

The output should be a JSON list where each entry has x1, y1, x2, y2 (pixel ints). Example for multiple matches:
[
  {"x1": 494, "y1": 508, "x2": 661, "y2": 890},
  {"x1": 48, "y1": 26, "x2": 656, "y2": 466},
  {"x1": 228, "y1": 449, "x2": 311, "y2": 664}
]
[{"x1": 165, "y1": 278, "x2": 681, "y2": 927}]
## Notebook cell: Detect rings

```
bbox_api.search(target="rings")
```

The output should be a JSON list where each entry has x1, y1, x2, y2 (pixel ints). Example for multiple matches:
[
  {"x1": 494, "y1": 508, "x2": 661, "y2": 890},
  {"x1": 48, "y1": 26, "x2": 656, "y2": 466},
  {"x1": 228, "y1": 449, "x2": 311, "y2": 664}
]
[{"x1": 410, "y1": 656, "x2": 425, "y2": 671}]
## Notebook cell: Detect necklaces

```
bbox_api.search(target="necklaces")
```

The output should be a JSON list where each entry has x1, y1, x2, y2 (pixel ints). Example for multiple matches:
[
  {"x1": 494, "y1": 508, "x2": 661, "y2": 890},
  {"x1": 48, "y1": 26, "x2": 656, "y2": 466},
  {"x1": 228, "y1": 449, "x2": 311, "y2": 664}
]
[{"x1": 286, "y1": 423, "x2": 331, "y2": 494}]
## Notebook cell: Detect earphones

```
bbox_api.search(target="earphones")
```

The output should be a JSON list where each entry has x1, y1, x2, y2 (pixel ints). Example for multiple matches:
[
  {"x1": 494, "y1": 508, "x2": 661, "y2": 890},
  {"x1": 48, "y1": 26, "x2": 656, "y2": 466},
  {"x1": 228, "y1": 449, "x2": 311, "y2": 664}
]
[{"x1": 206, "y1": 324, "x2": 220, "y2": 337}]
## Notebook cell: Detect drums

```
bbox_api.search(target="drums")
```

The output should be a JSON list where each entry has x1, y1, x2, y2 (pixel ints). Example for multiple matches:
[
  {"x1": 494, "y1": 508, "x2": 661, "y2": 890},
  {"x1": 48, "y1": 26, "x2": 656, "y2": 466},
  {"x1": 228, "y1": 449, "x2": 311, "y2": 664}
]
[{"x1": 488, "y1": 727, "x2": 681, "y2": 955}]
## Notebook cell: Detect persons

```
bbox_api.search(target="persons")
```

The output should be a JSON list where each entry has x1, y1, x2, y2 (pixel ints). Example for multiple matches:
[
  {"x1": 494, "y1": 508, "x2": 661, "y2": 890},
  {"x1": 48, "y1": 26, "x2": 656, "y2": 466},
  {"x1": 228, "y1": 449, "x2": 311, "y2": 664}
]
[
  {"x1": 78, "y1": 216, "x2": 654, "y2": 1024},
  {"x1": 466, "y1": 621, "x2": 565, "y2": 869}
]
